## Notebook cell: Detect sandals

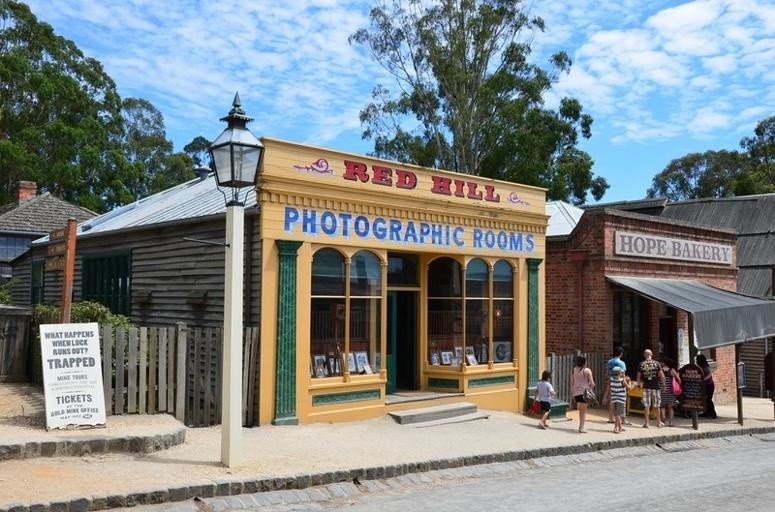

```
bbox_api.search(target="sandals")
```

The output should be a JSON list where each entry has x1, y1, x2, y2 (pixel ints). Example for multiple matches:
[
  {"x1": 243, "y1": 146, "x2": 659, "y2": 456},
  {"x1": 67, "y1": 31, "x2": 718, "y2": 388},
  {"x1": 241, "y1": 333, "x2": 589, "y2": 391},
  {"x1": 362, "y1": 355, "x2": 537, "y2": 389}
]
[{"x1": 538, "y1": 422, "x2": 548, "y2": 429}]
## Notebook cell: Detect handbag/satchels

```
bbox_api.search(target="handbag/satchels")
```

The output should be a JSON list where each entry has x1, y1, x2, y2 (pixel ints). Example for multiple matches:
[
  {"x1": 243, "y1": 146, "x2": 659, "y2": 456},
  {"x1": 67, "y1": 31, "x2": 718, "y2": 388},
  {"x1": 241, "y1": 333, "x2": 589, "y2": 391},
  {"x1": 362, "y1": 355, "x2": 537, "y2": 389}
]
[
  {"x1": 583, "y1": 388, "x2": 597, "y2": 404},
  {"x1": 672, "y1": 368, "x2": 682, "y2": 396},
  {"x1": 532, "y1": 401, "x2": 540, "y2": 413}
]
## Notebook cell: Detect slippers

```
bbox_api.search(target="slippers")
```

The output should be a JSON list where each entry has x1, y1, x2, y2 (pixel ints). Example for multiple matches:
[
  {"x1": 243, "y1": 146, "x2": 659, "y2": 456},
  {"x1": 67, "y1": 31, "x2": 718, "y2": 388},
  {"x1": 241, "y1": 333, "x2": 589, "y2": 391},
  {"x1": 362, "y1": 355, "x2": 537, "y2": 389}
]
[
  {"x1": 579, "y1": 429, "x2": 588, "y2": 433},
  {"x1": 607, "y1": 419, "x2": 673, "y2": 433}
]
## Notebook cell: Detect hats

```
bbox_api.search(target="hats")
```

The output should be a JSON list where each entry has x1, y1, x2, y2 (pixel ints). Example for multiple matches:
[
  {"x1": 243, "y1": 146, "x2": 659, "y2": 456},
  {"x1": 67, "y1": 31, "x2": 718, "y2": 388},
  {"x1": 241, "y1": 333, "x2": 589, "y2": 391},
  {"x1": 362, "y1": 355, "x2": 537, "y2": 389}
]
[{"x1": 613, "y1": 366, "x2": 624, "y2": 372}]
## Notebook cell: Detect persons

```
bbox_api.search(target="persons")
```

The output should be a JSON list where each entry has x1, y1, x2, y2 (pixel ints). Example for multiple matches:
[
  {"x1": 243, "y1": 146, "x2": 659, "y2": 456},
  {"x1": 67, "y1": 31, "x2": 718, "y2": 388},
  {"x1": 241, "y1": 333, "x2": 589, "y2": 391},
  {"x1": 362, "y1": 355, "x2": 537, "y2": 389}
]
[
  {"x1": 636, "y1": 347, "x2": 667, "y2": 428},
  {"x1": 695, "y1": 353, "x2": 717, "y2": 419},
  {"x1": 658, "y1": 358, "x2": 683, "y2": 427},
  {"x1": 571, "y1": 356, "x2": 596, "y2": 433},
  {"x1": 533, "y1": 370, "x2": 557, "y2": 429},
  {"x1": 606, "y1": 347, "x2": 633, "y2": 426},
  {"x1": 608, "y1": 366, "x2": 634, "y2": 433}
]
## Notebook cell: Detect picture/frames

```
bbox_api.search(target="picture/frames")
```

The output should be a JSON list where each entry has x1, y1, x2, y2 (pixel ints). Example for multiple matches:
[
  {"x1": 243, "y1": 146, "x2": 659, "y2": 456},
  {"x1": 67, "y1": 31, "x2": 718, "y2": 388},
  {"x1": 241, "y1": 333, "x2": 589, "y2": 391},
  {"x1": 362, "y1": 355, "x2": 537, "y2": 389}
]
[
  {"x1": 310, "y1": 350, "x2": 375, "y2": 378},
  {"x1": 430, "y1": 338, "x2": 513, "y2": 367}
]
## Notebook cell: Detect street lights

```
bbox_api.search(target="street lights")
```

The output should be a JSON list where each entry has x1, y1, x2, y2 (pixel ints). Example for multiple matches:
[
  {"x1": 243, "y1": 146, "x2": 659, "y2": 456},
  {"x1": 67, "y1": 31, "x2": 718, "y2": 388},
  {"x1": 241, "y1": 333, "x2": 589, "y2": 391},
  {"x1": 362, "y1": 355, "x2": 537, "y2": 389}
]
[{"x1": 210, "y1": 92, "x2": 265, "y2": 472}]
